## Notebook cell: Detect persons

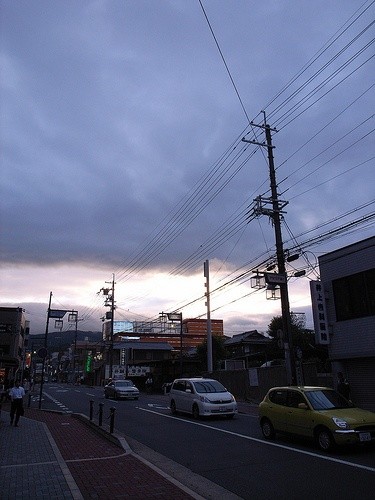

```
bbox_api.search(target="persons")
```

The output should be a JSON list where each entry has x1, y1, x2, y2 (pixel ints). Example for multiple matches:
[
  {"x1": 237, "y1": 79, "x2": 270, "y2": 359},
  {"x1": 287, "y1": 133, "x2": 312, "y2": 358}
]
[
  {"x1": 9, "y1": 379, "x2": 25, "y2": 427},
  {"x1": 337, "y1": 371, "x2": 351, "y2": 402}
]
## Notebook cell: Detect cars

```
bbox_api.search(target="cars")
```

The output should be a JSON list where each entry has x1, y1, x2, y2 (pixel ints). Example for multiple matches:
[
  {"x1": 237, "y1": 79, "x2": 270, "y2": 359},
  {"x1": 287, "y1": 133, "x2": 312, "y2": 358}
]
[
  {"x1": 258, "y1": 385, "x2": 375, "y2": 453},
  {"x1": 104, "y1": 380, "x2": 140, "y2": 400},
  {"x1": 169, "y1": 378, "x2": 238, "y2": 420}
]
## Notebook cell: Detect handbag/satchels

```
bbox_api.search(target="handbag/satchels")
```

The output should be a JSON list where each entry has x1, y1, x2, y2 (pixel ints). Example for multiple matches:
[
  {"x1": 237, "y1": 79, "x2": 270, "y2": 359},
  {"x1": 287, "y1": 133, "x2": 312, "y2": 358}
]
[{"x1": 20, "y1": 408, "x2": 24, "y2": 416}]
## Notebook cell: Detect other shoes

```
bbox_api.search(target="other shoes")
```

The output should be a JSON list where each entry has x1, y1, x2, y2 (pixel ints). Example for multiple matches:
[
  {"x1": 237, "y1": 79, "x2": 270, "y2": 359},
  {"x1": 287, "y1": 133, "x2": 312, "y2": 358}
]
[
  {"x1": 10, "y1": 422, "x2": 13, "y2": 426},
  {"x1": 14, "y1": 424, "x2": 19, "y2": 427}
]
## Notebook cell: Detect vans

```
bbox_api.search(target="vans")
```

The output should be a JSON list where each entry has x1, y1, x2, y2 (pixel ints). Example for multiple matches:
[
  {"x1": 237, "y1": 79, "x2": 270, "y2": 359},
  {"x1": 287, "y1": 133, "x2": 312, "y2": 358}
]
[
  {"x1": 44, "y1": 377, "x2": 49, "y2": 383},
  {"x1": 52, "y1": 377, "x2": 56, "y2": 382}
]
